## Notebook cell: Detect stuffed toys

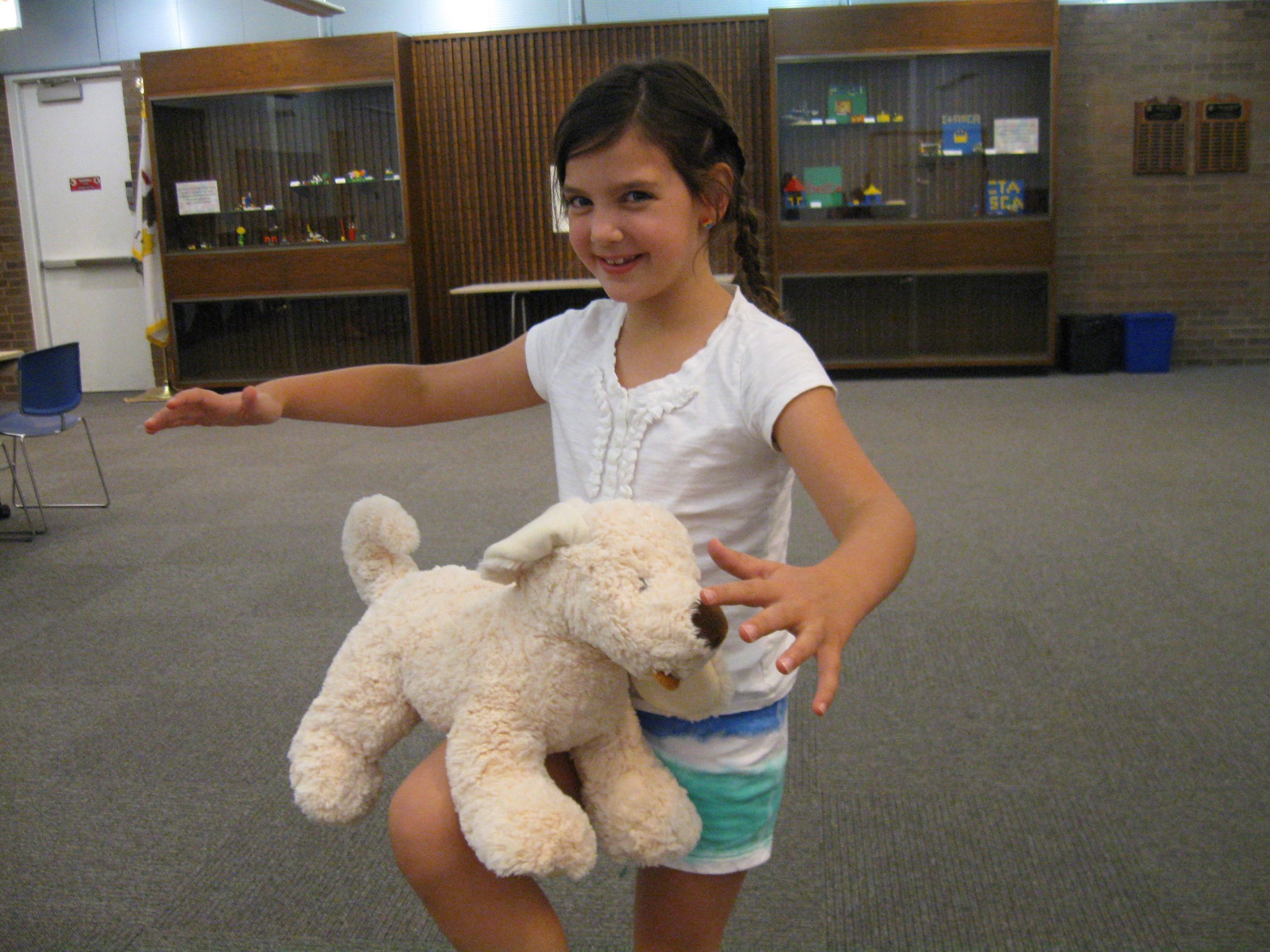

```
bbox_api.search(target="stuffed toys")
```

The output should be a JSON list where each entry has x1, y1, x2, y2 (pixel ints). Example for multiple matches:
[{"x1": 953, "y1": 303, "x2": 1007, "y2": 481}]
[{"x1": 287, "y1": 493, "x2": 731, "y2": 881}]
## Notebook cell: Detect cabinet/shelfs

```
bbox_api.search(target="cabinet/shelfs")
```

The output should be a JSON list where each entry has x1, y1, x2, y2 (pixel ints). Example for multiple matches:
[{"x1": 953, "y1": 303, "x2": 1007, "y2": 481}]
[
  {"x1": 770, "y1": 0, "x2": 1058, "y2": 369},
  {"x1": 141, "y1": 31, "x2": 420, "y2": 393}
]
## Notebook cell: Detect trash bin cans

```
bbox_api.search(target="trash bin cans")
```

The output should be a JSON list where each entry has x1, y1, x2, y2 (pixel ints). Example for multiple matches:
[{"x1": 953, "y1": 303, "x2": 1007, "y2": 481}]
[
  {"x1": 1058, "y1": 314, "x2": 1119, "y2": 373},
  {"x1": 1119, "y1": 312, "x2": 1177, "y2": 373}
]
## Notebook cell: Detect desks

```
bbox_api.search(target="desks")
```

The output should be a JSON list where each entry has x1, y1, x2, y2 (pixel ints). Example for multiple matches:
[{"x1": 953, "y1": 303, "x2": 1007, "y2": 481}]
[{"x1": 449, "y1": 274, "x2": 737, "y2": 344}]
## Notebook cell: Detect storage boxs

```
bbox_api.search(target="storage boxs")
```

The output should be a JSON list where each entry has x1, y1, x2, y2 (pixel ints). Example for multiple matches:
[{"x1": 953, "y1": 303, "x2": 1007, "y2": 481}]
[{"x1": 1065, "y1": 311, "x2": 1176, "y2": 374}]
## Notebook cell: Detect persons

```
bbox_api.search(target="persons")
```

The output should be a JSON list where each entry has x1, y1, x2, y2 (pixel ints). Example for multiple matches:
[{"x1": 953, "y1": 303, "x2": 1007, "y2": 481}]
[{"x1": 143, "y1": 60, "x2": 917, "y2": 952}]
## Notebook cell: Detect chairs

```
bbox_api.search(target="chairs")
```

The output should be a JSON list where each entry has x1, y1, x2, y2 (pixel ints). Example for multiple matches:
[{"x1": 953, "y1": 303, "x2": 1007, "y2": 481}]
[{"x1": 0, "y1": 341, "x2": 110, "y2": 543}]
